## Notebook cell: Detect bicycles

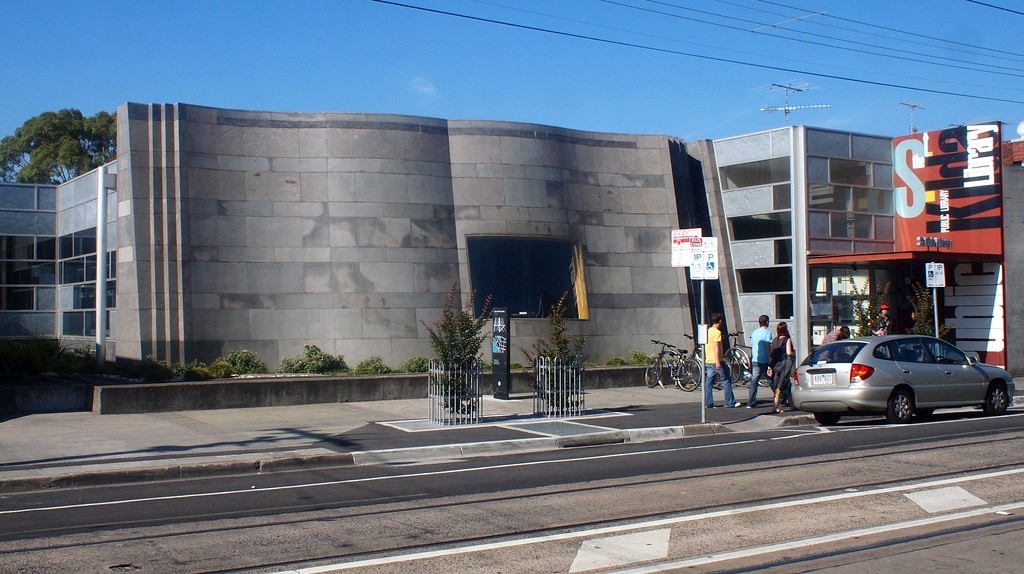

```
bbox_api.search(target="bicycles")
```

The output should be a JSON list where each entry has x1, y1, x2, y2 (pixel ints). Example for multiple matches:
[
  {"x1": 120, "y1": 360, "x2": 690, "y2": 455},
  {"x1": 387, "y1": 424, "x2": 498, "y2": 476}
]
[{"x1": 644, "y1": 331, "x2": 770, "y2": 393}]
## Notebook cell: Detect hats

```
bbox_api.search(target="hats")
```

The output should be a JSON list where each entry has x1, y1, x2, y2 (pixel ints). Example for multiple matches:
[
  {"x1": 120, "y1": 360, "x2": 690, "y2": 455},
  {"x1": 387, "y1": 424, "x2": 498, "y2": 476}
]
[{"x1": 879, "y1": 304, "x2": 888, "y2": 308}]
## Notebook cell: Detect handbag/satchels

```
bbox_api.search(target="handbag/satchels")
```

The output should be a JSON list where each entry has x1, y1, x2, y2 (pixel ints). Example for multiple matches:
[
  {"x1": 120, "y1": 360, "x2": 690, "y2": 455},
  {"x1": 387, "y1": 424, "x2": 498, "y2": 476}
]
[{"x1": 771, "y1": 337, "x2": 789, "y2": 372}]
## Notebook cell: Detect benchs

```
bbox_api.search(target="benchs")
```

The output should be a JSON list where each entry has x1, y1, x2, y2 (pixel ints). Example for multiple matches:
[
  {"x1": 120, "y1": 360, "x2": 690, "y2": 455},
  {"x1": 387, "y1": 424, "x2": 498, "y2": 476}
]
[{"x1": 838, "y1": 351, "x2": 850, "y2": 361}]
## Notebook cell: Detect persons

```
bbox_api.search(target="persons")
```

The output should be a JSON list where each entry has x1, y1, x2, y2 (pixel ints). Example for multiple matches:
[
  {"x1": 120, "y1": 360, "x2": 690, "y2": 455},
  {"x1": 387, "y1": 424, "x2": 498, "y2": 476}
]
[
  {"x1": 820, "y1": 326, "x2": 850, "y2": 362},
  {"x1": 871, "y1": 304, "x2": 892, "y2": 336},
  {"x1": 706, "y1": 313, "x2": 743, "y2": 407},
  {"x1": 767, "y1": 322, "x2": 795, "y2": 413},
  {"x1": 746, "y1": 315, "x2": 788, "y2": 408},
  {"x1": 911, "y1": 310, "x2": 919, "y2": 328}
]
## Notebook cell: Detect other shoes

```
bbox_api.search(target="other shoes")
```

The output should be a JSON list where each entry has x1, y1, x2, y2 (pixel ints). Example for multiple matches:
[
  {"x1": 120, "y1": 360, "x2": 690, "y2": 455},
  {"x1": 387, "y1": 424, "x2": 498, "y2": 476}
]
[
  {"x1": 724, "y1": 402, "x2": 740, "y2": 408},
  {"x1": 746, "y1": 405, "x2": 759, "y2": 409},
  {"x1": 705, "y1": 405, "x2": 719, "y2": 409}
]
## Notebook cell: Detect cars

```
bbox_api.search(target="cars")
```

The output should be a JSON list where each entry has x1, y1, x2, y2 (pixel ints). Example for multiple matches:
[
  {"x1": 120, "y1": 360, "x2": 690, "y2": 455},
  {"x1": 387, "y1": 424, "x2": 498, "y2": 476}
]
[{"x1": 789, "y1": 334, "x2": 1016, "y2": 425}]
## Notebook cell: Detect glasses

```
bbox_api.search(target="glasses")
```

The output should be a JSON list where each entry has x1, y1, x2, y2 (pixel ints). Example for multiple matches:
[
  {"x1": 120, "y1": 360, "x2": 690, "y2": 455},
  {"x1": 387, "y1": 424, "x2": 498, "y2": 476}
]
[{"x1": 882, "y1": 308, "x2": 888, "y2": 310}]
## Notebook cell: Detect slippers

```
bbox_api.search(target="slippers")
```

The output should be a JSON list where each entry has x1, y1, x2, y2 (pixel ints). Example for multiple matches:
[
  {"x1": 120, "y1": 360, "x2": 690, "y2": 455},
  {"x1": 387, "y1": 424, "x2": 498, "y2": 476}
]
[
  {"x1": 791, "y1": 407, "x2": 798, "y2": 411},
  {"x1": 772, "y1": 409, "x2": 784, "y2": 413}
]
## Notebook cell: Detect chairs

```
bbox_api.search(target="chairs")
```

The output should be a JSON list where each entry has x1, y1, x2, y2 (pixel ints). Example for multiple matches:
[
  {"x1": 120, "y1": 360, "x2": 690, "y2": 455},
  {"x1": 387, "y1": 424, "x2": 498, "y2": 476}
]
[{"x1": 901, "y1": 349, "x2": 918, "y2": 364}]
